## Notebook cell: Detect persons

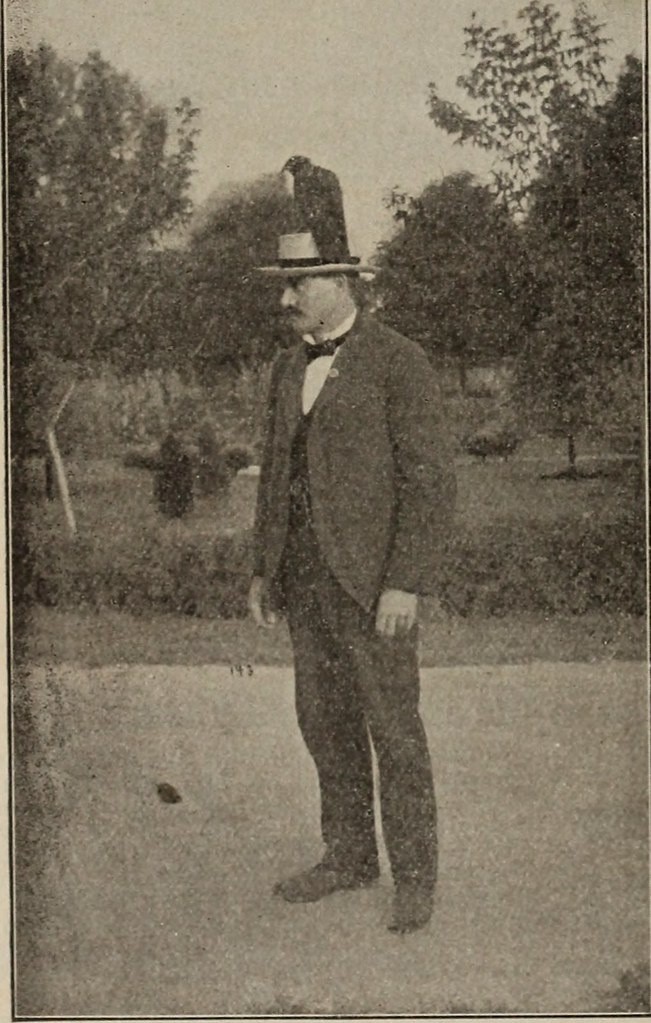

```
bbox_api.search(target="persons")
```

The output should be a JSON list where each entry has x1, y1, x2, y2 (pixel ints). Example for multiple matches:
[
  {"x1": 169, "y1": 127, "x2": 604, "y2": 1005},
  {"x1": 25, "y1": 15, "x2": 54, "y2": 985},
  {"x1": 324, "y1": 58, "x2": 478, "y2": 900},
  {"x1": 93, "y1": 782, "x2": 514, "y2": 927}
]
[
  {"x1": 152, "y1": 436, "x2": 195, "y2": 518},
  {"x1": 245, "y1": 233, "x2": 458, "y2": 932}
]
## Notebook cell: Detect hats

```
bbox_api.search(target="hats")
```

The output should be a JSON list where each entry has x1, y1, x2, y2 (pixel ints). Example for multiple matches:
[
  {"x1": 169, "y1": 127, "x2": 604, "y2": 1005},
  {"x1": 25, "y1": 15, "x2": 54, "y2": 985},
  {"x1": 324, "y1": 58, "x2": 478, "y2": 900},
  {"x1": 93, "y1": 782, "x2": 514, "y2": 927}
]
[{"x1": 253, "y1": 231, "x2": 383, "y2": 277}]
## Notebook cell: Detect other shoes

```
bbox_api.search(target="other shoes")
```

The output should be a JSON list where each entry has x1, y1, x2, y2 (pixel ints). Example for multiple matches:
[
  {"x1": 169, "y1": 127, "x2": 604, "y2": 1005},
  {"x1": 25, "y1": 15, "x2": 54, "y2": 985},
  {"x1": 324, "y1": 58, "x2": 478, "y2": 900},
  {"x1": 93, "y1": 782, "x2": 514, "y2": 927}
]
[
  {"x1": 387, "y1": 883, "x2": 433, "y2": 933},
  {"x1": 274, "y1": 864, "x2": 374, "y2": 903}
]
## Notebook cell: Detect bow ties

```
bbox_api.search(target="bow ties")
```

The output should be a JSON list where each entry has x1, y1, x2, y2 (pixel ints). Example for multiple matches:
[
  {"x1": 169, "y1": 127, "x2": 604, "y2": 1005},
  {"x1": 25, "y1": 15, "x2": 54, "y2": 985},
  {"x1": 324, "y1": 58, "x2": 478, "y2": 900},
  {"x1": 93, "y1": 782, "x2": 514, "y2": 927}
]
[{"x1": 306, "y1": 329, "x2": 351, "y2": 365}]
